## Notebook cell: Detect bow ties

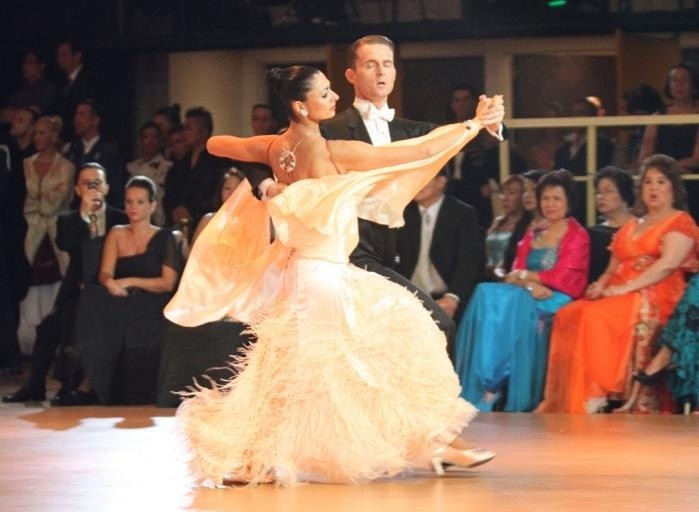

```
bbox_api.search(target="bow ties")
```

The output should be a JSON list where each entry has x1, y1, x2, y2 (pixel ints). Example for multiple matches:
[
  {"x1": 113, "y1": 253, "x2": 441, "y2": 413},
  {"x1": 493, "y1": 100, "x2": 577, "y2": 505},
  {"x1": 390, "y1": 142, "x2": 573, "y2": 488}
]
[{"x1": 367, "y1": 102, "x2": 396, "y2": 122}]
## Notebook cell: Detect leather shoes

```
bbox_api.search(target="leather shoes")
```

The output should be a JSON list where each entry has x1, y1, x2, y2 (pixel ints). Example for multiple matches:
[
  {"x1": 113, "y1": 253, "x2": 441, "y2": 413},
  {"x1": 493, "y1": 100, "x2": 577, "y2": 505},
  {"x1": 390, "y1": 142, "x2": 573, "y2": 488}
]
[
  {"x1": 52, "y1": 383, "x2": 98, "y2": 406},
  {"x1": 3, "y1": 381, "x2": 45, "y2": 402},
  {"x1": 222, "y1": 468, "x2": 276, "y2": 484}
]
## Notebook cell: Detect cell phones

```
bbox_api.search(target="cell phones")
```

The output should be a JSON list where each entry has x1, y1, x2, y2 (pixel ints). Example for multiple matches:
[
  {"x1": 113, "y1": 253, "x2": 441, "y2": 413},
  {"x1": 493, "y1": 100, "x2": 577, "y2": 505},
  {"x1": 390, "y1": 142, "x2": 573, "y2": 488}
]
[{"x1": 88, "y1": 182, "x2": 97, "y2": 189}]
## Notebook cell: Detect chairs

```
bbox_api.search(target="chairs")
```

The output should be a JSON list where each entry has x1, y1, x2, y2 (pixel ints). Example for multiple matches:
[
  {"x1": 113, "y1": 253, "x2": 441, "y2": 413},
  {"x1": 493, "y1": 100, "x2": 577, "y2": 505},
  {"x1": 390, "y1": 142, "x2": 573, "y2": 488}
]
[{"x1": 585, "y1": 225, "x2": 621, "y2": 284}]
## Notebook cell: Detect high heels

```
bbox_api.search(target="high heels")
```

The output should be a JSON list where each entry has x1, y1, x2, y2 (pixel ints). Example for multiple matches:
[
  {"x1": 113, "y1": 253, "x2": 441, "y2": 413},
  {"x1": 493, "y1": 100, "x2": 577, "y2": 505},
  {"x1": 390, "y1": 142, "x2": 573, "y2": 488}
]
[
  {"x1": 428, "y1": 441, "x2": 497, "y2": 477},
  {"x1": 633, "y1": 361, "x2": 679, "y2": 391}
]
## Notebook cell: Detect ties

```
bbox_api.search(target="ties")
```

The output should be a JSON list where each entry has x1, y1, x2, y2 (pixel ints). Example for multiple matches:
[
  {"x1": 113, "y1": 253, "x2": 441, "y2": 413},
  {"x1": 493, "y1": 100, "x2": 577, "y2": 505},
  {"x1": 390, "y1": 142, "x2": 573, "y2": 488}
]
[{"x1": 417, "y1": 212, "x2": 436, "y2": 293}]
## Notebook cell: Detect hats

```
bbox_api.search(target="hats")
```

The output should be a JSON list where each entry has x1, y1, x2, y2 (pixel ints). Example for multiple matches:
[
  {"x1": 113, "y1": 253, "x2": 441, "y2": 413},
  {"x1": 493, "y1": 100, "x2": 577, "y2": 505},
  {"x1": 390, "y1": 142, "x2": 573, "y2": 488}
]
[{"x1": 474, "y1": 390, "x2": 504, "y2": 414}]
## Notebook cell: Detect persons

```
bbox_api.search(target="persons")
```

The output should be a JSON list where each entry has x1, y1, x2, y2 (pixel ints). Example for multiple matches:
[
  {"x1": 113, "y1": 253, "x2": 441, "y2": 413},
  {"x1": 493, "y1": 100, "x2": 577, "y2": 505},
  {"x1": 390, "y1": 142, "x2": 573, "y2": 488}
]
[
  {"x1": 388, "y1": 60, "x2": 698, "y2": 416},
  {"x1": 239, "y1": 34, "x2": 509, "y2": 368},
  {"x1": 2, "y1": 41, "x2": 277, "y2": 406},
  {"x1": 172, "y1": 66, "x2": 505, "y2": 486}
]
